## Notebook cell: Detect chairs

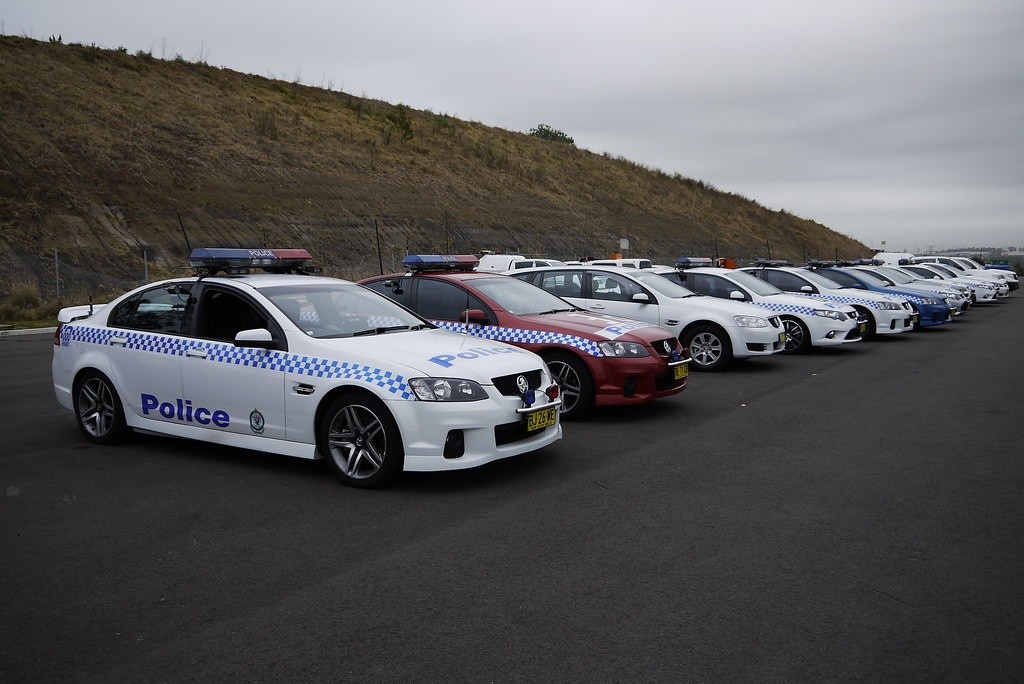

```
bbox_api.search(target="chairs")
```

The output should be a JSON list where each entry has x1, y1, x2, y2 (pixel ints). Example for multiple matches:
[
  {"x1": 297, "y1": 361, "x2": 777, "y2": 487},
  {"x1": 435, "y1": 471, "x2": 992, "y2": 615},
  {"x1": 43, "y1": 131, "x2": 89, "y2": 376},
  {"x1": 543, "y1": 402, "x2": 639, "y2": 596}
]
[
  {"x1": 268, "y1": 298, "x2": 301, "y2": 330},
  {"x1": 590, "y1": 278, "x2": 622, "y2": 302}
]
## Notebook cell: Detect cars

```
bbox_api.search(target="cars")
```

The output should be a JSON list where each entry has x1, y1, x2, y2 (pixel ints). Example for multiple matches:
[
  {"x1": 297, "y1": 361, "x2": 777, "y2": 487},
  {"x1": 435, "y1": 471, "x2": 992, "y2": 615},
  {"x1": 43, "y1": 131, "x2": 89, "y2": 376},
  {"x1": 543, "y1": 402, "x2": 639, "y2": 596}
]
[
  {"x1": 52, "y1": 249, "x2": 562, "y2": 488},
  {"x1": 646, "y1": 258, "x2": 866, "y2": 355},
  {"x1": 359, "y1": 255, "x2": 691, "y2": 422},
  {"x1": 854, "y1": 265, "x2": 969, "y2": 317},
  {"x1": 873, "y1": 253, "x2": 1018, "y2": 308},
  {"x1": 798, "y1": 261, "x2": 957, "y2": 331},
  {"x1": 501, "y1": 265, "x2": 785, "y2": 373},
  {"x1": 736, "y1": 260, "x2": 919, "y2": 340}
]
